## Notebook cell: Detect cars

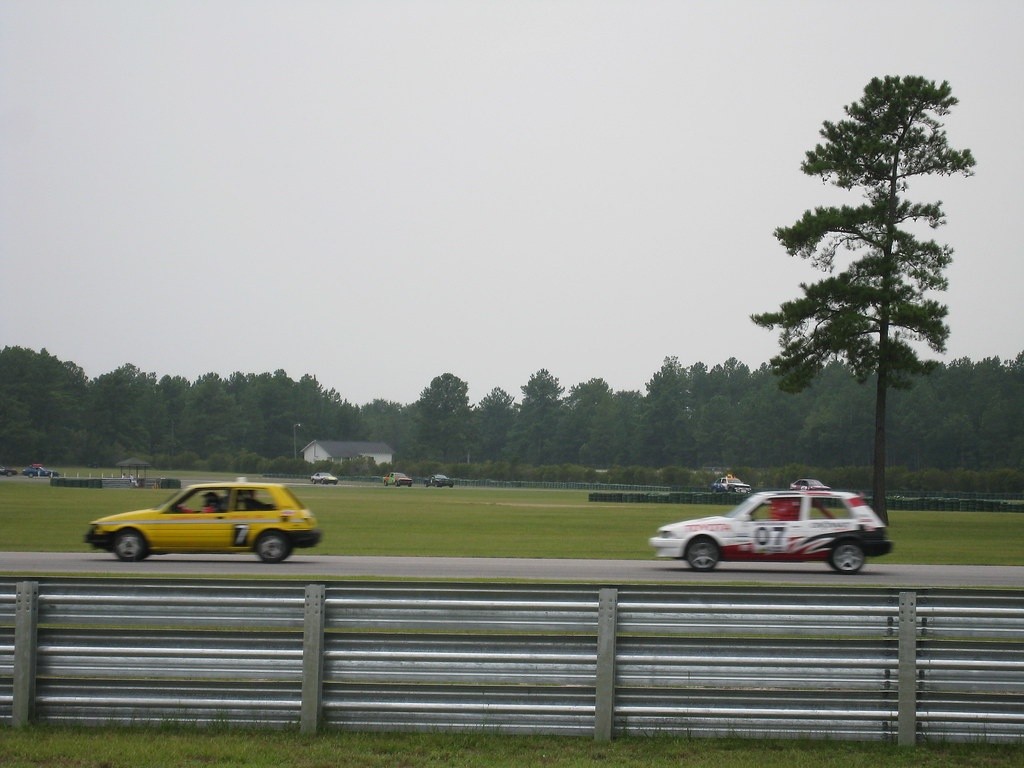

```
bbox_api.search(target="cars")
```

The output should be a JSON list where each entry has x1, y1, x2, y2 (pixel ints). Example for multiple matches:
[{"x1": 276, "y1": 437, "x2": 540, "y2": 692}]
[
  {"x1": 0, "y1": 465, "x2": 18, "y2": 478},
  {"x1": 85, "y1": 477, "x2": 323, "y2": 564},
  {"x1": 22, "y1": 464, "x2": 60, "y2": 478},
  {"x1": 712, "y1": 473, "x2": 752, "y2": 494},
  {"x1": 789, "y1": 478, "x2": 833, "y2": 492},
  {"x1": 310, "y1": 472, "x2": 339, "y2": 486},
  {"x1": 425, "y1": 474, "x2": 455, "y2": 488},
  {"x1": 382, "y1": 472, "x2": 414, "y2": 487},
  {"x1": 647, "y1": 489, "x2": 895, "y2": 578}
]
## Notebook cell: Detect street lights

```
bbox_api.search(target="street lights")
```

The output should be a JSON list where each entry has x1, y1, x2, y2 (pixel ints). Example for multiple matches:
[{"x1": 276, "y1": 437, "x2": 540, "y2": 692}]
[{"x1": 293, "y1": 423, "x2": 302, "y2": 459}]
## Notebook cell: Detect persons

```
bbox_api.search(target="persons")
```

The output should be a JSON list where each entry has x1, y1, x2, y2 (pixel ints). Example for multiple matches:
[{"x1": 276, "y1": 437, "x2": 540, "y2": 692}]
[
  {"x1": 130, "y1": 473, "x2": 138, "y2": 487},
  {"x1": 122, "y1": 474, "x2": 124, "y2": 479},
  {"x1": 176, "y1": 492, "x2": 219, "y2": 512},
  {"x1": 772, "y1": 499, "x2": 799, "y2": 519}
]
